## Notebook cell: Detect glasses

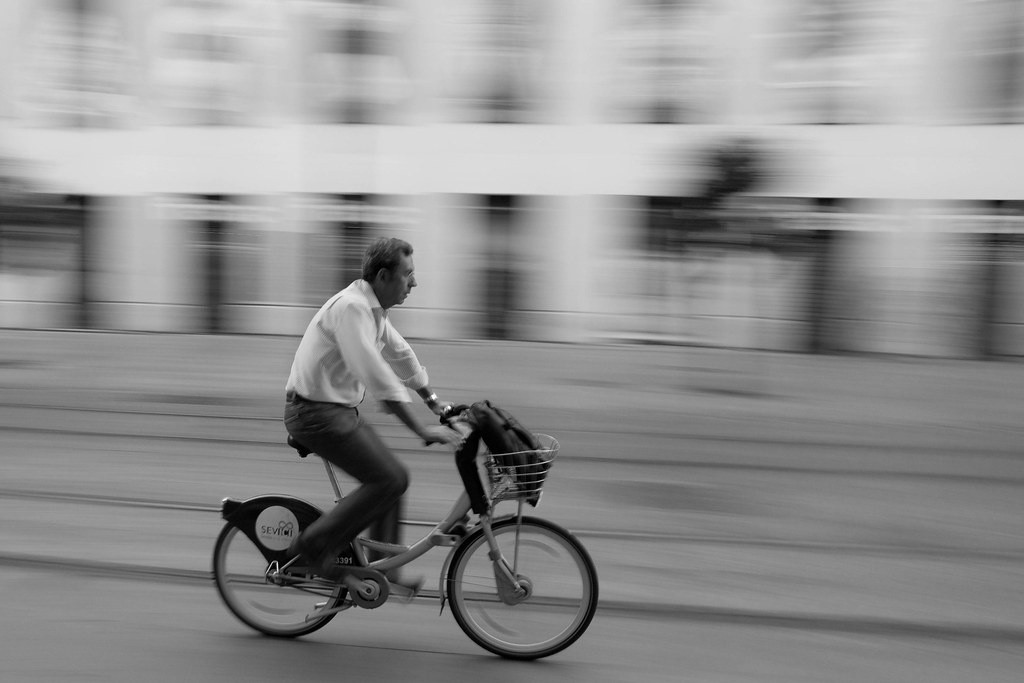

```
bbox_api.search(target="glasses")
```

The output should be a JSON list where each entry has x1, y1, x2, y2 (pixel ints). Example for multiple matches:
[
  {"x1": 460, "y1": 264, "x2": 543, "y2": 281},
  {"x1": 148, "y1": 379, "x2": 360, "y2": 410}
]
[{"x1": 385, "y1": 267, "x2": 414, "y2": 278}]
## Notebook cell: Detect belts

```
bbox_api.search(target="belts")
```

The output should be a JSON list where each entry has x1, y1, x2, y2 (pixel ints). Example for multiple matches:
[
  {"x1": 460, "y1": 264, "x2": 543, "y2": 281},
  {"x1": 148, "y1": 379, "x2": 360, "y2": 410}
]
[{"x1": 284, "y1": 391, "x2": 312, "y2": 403}]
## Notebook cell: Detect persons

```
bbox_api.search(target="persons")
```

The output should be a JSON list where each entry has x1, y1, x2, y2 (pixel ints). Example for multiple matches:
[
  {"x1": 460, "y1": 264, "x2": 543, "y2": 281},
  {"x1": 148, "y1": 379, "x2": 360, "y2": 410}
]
[{"x1": 279, "y1": 238, "x2": 463, "y2": 600}]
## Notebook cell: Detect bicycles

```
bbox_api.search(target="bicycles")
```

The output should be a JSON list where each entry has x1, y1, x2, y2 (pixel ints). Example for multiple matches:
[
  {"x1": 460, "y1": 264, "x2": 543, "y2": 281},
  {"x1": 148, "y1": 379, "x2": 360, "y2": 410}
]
[{"x1": 209, "y1": 407, "x2": 601, "y2": 660}]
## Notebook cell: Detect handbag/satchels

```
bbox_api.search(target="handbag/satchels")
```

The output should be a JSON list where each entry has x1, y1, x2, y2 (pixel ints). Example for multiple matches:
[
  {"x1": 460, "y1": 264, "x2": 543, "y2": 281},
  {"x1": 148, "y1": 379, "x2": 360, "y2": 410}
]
[{"x1": 466, "y1": 400, "x2": 552, "y2": 506}]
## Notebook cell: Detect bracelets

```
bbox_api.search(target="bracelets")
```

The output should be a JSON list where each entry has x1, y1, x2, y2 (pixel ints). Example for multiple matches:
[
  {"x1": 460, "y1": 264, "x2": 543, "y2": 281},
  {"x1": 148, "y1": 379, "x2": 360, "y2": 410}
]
[{"x1": 423, "y1": 393, "x2": 438, "y2": 403}]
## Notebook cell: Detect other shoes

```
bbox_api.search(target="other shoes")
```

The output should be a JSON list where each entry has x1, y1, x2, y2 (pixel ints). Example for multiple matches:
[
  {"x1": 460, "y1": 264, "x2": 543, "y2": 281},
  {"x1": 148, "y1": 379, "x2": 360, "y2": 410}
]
[
  {"x1": 287, "y1": 534, "x2": 337, "y2": 569},
  {"x1": 382, "y1": 565, "x2": 420, "y2": 589}
]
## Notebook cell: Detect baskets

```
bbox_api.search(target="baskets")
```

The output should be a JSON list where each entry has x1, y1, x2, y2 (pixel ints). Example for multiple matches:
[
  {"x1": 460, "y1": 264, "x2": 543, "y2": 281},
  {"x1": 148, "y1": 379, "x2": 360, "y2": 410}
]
[{"x1": 484, "y1": 432, "x2": 560, "y2": 501}]
